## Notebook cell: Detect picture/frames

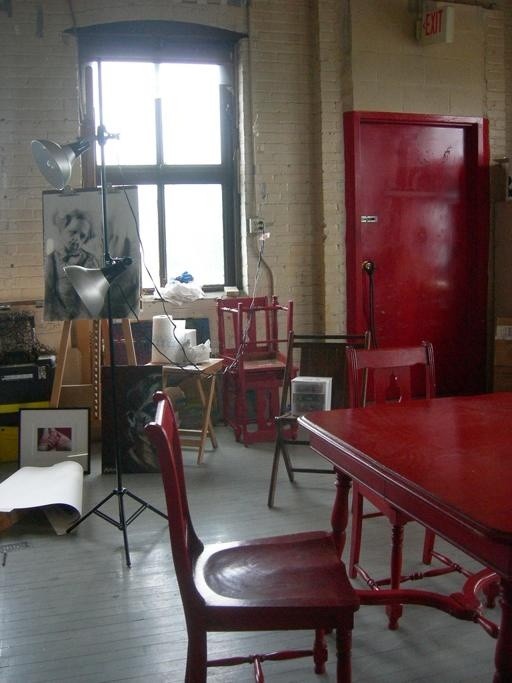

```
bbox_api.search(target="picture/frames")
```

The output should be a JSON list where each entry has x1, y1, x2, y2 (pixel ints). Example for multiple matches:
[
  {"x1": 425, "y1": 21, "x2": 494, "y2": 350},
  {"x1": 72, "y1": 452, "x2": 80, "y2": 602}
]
[
  {"x1": 100, "y1": 364, "x2": 164, "y2": 475},
  {"x1": 41, "y1": 184, "x2": 142, "y2": 319},
  {"x1": 17, "y1": 407, "x2": 91, "y2": 475}
]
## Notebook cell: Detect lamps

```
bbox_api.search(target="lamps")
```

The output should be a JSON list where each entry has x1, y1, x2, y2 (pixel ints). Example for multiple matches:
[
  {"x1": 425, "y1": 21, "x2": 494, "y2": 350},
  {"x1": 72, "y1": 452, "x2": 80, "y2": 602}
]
[{"x1": 29, "y1": 55, "x2": 170, "y2": 569}]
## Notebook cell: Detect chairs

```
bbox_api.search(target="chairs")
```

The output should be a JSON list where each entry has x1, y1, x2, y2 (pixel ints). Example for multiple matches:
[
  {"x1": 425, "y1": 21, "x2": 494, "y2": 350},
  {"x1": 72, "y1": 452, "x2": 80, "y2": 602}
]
[
  {"x1": 266, "y1": 330, "x2": 372, "y2": 508},
  {"x1": 143, "y1": 388, "x2": 361, "y2": 683},
  {"x1": 345, "y1": 339, "x2": 437, "y2": 631}
]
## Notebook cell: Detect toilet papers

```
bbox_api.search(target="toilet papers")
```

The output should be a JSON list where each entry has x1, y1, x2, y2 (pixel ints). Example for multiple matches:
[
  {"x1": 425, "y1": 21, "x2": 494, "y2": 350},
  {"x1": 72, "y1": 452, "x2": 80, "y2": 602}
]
[{"x1": 152, "y1": 315, "x2": 173, "y2": 363}]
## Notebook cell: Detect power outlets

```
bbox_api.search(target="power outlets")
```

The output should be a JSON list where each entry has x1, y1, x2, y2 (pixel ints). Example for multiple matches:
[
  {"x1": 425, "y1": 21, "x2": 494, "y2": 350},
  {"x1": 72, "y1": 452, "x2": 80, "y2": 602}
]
[{"x1": 249, "y1": 216, "x2": 266, "y2": 235}]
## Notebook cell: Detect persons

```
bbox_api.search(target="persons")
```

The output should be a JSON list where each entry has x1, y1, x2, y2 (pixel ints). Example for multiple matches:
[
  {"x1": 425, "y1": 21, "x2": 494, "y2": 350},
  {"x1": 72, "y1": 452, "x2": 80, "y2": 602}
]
[{"x1": 44, "y1": 208, "x2": 101, "y2": 320}]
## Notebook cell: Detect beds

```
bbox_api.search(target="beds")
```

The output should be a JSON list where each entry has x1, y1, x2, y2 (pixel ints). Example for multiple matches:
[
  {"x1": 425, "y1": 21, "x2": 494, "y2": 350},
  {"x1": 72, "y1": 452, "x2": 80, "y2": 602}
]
[{"x1": 216, "y1": 296, "x2": 299, "y2": 447}]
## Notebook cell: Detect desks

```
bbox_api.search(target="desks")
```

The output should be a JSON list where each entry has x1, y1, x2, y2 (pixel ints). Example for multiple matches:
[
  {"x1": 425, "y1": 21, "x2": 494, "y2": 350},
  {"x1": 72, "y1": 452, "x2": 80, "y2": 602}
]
[
  {"x1": 297, "y1": 389, "x2": 512, "y2": 683},
  {"x1": 144, "y1": 357, "x2": 226, "y2": 465}
]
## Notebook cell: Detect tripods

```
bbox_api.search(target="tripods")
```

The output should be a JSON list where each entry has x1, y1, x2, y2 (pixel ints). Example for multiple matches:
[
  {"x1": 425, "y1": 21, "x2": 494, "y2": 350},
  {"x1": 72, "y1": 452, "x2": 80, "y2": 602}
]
[{"x1": 65, "y1": 316, "x2": 169, "y2": 570}]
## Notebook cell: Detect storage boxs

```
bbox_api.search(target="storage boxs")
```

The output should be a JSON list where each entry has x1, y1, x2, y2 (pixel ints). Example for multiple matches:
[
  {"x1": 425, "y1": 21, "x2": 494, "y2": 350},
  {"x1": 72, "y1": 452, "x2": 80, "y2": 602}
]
[{"x1": 493, "y1": 201, "x2": 512, "y2": 391}]
